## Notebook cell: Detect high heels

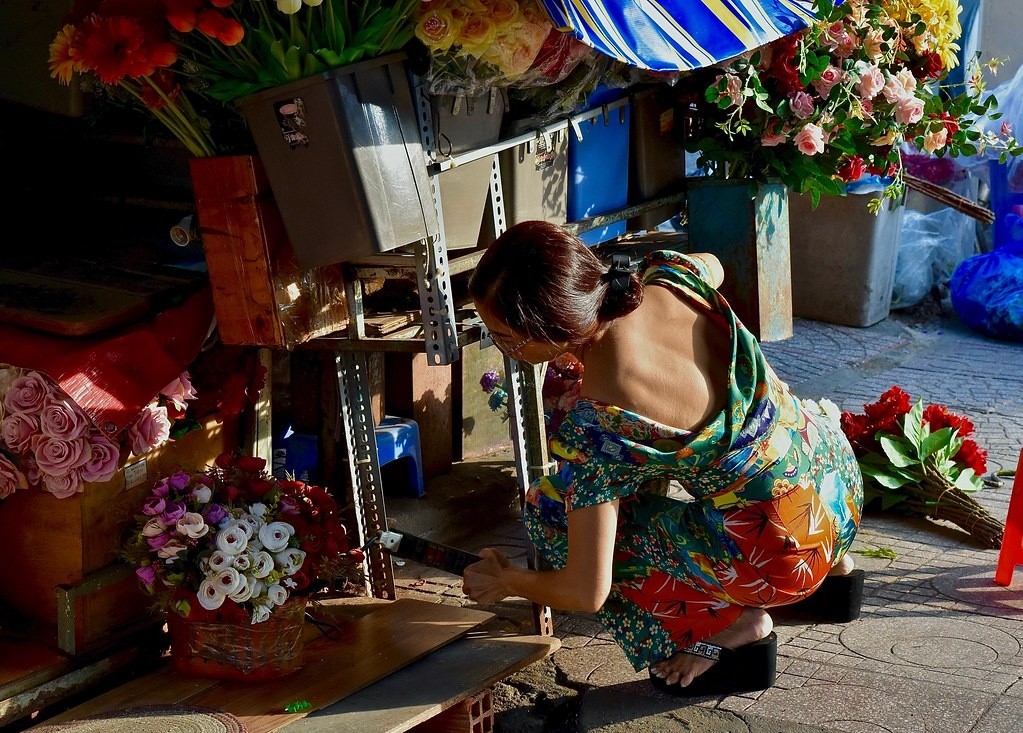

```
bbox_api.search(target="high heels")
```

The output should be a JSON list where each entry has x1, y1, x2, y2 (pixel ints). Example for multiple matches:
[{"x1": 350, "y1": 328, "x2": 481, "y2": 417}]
[
  {"x1": 648, "y1": 631, "x2": 777, "y2": 698},
  {"x1": 772, "y1": 570, "x2": 869, "y2": 624}
]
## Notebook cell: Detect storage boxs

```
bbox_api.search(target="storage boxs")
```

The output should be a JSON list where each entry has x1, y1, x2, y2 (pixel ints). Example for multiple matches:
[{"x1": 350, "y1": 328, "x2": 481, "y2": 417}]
[{"x1": 2, "y1": 416, "x2": 228, "y2": 641}]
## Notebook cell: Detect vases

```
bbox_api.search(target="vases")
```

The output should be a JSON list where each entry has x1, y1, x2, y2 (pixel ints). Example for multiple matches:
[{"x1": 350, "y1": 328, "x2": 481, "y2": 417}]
[
  {"x1": 185, "y1": 53, "x2": 985, "y2": 493},
  {"x1": 163, "y1": 587, "x2": 312, "y2": 680}
]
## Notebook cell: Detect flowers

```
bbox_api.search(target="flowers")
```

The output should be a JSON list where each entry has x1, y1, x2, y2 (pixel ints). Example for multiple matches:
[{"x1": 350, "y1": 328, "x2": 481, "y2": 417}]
[
  {"x1": 47, "y1": 0, "x2": 1023, "y2": 214},
  {"x1": 0, "y1": 362, "x2": 195, "y2": 502},
  {"x1": 838, "y1": 386, "x2": 1007, "y2": 550},
  {"x1": 110, "y1": 451, "x2": 366, "y2": 627}
]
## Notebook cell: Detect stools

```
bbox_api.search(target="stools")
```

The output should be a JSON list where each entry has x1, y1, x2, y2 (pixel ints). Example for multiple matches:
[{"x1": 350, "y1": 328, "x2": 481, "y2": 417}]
[{"x1": 273, "y1": 414, "x2": 425, "y2": 505}]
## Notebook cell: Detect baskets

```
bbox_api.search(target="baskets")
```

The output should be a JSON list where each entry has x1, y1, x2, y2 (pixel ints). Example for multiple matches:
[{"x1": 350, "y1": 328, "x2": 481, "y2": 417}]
[{"x1": 155, "y1": 581, "x2": 307, "y2": 680}]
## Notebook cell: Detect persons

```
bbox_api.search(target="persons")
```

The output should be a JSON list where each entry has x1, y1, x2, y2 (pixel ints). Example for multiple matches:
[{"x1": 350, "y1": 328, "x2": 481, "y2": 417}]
[{"x1": 462, "y1": 220, "x2": 864, "y2": 700}]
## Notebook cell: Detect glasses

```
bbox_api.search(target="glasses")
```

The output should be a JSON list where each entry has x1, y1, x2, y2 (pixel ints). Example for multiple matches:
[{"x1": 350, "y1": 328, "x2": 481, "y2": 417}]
[{"x1": 486, "y1": 330, "x2": 532, "y2": 361}]
